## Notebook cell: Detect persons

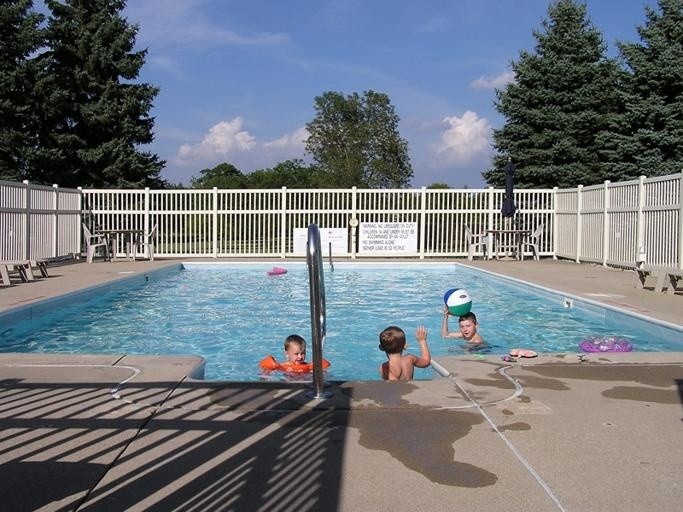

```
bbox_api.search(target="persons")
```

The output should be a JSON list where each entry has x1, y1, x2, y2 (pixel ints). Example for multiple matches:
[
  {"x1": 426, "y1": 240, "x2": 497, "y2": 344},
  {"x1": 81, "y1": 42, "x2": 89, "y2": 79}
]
[
  {"x1": 282, "y1": 335, "x2": 310, "y2": 363},
  {"x1": 440, "y1": 305, "x2": 490, "y2": 351},
  {"x1": 372, "y1": 324, "x2": 433, "y2": 382}
]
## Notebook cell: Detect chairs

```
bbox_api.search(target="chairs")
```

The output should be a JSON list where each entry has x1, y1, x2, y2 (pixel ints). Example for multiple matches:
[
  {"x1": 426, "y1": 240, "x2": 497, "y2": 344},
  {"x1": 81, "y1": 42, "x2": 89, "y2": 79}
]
[
  {"x1": 520, "y1": 224, "x2": 544, "y2": 261},
  {"x1": 81, "y1": 222, "x2": 110, "y2": 263},
  {"x1": 463, "y1": 224, "x2": 487, "y2": 261},
  {"x1": 132, "y1": 223, "x2": 160, "y2": 261}
]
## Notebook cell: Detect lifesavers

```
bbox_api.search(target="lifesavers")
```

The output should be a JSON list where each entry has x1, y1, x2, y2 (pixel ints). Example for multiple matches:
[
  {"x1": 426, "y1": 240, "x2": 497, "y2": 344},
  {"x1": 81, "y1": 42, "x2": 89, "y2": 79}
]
[
  {"x1": 579, "y1": 335, "x2": 633, "y2": 351},
  {"x1": 259, "y1": 356, "x2": 330, "y2": 372}
]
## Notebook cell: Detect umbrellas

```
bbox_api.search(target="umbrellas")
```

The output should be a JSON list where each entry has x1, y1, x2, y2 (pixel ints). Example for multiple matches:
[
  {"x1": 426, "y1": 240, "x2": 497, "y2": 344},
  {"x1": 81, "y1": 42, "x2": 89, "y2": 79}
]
[{"x1": 499, "y1": 153, "x2": 521, "y2": 263}]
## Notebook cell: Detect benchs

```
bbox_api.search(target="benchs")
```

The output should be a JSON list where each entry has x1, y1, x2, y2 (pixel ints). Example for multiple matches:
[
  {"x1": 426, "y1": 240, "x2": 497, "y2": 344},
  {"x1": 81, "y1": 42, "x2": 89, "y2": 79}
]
[
  {"x1": 634, "y1": 264, "x2": 682, "y2": 295},
  {"x1": 0, "y1": 258, "x2": 49, "y2": 286}
]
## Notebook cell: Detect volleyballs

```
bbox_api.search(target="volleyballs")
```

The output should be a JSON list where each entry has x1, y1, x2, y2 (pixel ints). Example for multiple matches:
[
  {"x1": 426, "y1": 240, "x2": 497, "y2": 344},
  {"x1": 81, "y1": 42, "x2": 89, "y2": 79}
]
[{"x1": 444, "y1": 288, "x2": 471, "y2": 316}]
